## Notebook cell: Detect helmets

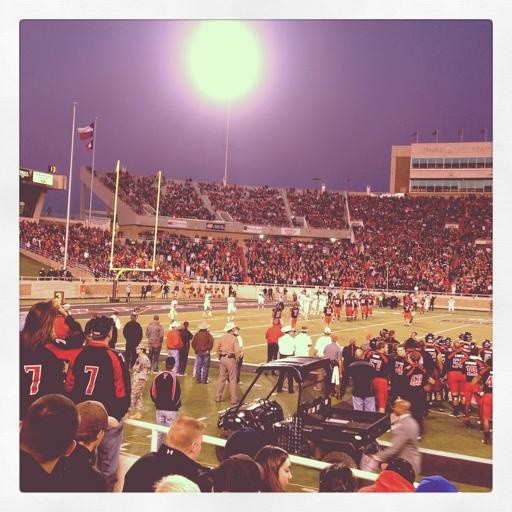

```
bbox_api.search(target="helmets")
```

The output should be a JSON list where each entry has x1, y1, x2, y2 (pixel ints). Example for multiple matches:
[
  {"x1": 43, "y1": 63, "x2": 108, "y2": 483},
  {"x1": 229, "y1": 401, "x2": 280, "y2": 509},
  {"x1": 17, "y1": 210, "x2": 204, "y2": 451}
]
[{"x1": 369, "y1": 329, "x2": 491, "y2": 350}]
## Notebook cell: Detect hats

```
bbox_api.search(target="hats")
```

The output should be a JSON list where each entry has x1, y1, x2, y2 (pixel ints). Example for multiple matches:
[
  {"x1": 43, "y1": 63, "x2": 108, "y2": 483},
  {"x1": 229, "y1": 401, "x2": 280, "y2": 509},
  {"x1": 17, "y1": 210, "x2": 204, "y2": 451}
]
[
  {"x1": 224, "y1": 322, "x2": 236, "y2": 332},
  {"x1": 416, "y1": 475, "x2": 457, "y2": 492},
  {"x1": 199, "y1": 323, "x2": 210, "y2": 329},
  {"x1": 92, "y1": 318, "x2": 113, "y2": 335},
  {"x1": 77, "y1": 400, "x2": 120, "y2": 435},
  {"x1": 357, "y1": 469, "x2": 416, "y2": 492},
  {"x1": 281, "y1": 325, "x2": 294, "y2": 333}
]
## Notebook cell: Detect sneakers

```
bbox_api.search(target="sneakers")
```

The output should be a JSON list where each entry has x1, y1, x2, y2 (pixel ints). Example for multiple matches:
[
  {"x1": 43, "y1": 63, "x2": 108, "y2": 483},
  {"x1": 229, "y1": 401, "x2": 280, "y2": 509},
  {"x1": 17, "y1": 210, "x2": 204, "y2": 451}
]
[{"x1": 130, "y1": 411, "x2": 142, "y2": 419}]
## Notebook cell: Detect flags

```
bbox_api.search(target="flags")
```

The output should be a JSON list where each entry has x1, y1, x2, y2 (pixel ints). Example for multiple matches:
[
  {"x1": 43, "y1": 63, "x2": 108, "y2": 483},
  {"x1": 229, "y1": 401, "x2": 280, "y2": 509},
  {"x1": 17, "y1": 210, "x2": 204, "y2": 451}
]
[
  {"x1": 432, "y1": 130, "x2": 437, "y2": 135},
  {"x1": 481, "y1": 128, "x2": 485, "y2": 133},
  {"x1": 85, "y1": 141, "x2": 93, "y2": 150},
  {"x1": 458, "y1": 129, "x2": 462, "y2": 135},
  {"x1": 77, "y1": 121, "x2": 93, "y2": 140},
  {"x1": 411, "y1": 131, "x2": 417, "y2": 136}
]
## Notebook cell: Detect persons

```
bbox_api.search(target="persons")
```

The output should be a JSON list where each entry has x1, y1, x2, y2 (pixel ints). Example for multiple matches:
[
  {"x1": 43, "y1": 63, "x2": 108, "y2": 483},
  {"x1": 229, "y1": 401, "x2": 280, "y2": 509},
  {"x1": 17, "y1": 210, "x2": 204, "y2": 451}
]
[
  {"x1": 144, "y1": 311, "x2": 492, "y2": 447},
  {"x1": 19, "y1": 167, "x2": 493, "y2": 326},
  {"x1": 124, "y1": 398, "x2": 456, "y2": 493},
  {"x1": 21, "y1": 298, "x2": 152, "y2": 492}
]
